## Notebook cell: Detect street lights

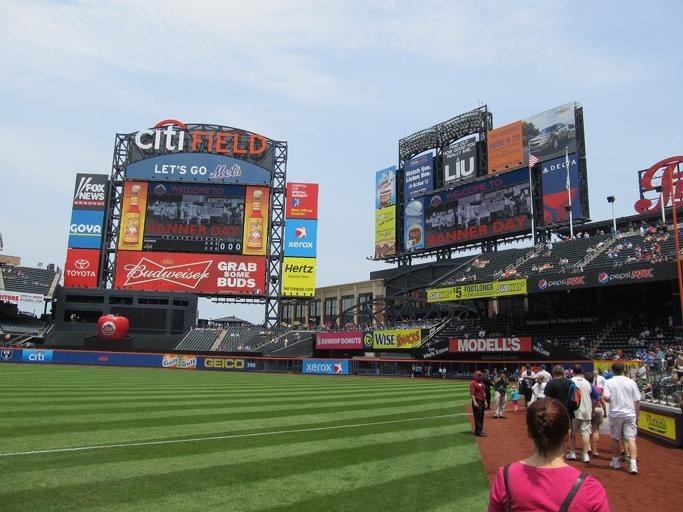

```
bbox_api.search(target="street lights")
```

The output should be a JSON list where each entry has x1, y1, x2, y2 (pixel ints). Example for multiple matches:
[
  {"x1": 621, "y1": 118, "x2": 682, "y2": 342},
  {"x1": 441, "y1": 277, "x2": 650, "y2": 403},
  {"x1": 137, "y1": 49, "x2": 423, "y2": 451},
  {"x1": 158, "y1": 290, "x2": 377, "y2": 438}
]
[
  {"x1": 654, "y1": 186, "x2": 667, "y2": 223},
  {"x1": 564, "y1": 204, "x2": 574, "y2": 240},
  {"x1": 606, "y1": 195, "x2": 620, "y2": 233},
  {"x1": 525, "y1": 210, "x2": 536, "y2": 246}
]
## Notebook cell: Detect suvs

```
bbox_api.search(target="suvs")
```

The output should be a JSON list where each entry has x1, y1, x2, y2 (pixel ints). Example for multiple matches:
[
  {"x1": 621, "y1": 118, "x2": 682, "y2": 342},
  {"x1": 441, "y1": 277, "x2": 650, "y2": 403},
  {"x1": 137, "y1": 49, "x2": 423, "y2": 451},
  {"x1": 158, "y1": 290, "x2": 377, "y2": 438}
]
[{"x1": 528, "y1": 121, "x2": 570, "y2": 155}]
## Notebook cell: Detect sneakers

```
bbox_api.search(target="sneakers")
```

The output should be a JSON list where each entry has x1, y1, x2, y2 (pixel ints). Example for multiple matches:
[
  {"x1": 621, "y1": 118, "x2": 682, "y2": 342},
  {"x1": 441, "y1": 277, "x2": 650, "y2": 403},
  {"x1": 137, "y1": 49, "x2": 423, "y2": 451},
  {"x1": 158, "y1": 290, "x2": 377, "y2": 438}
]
[
  {"x1": 565, "y1": 451, "x2": 576, "y2": 460},
  {"x1": 609, "y1": 460, "x2": 621, "y2": 469},
  {"x1": 629, "y1": 464, "x2": 638, "y2": 473},
  {"x1": 474, "y1": 430, "x2": 486, "y2": 437},
  {"x1": 578, "y1": 453, "x2": 590, "y2": 462}
]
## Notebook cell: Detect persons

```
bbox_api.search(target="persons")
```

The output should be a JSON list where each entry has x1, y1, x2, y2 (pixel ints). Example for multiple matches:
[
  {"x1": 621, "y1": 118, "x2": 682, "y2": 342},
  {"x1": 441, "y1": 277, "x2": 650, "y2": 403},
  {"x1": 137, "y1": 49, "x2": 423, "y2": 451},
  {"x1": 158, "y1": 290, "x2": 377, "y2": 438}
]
[
  {"x1": 0, "y1": 261, "x2": 53, "y2": 349},
  {"x1": 191, "y1": 316, "x2": 411, "y2": 356},
  {"x1": 486, "y1": 396, "x2": 610, "y2": 512},
  {"x1": 411, "y1": 214, "x2": 682, "y2": 475}
]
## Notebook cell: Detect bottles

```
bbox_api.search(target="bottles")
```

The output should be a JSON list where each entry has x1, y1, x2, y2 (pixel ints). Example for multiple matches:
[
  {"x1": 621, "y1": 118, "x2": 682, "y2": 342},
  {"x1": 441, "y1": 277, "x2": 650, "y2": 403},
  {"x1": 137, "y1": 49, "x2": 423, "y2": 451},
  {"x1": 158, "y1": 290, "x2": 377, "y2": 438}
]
[
  {"x1": 122, "y1": 185, "x2": 142, "y2": 246},
  {"x1": 244, "y1": 190, "x2": 264, "y2": 250}
]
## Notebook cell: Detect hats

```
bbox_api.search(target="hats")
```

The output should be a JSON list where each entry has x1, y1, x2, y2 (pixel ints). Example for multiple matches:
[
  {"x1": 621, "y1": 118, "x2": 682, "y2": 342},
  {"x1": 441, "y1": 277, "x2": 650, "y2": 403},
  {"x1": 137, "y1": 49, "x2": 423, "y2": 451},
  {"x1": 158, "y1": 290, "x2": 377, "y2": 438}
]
[
  {"x1": 539, "y1": 364, "x2": 546, "y2": 369},
  {"x1": 474, "y1": 370, "x2": 482, "y2": 376}
]
